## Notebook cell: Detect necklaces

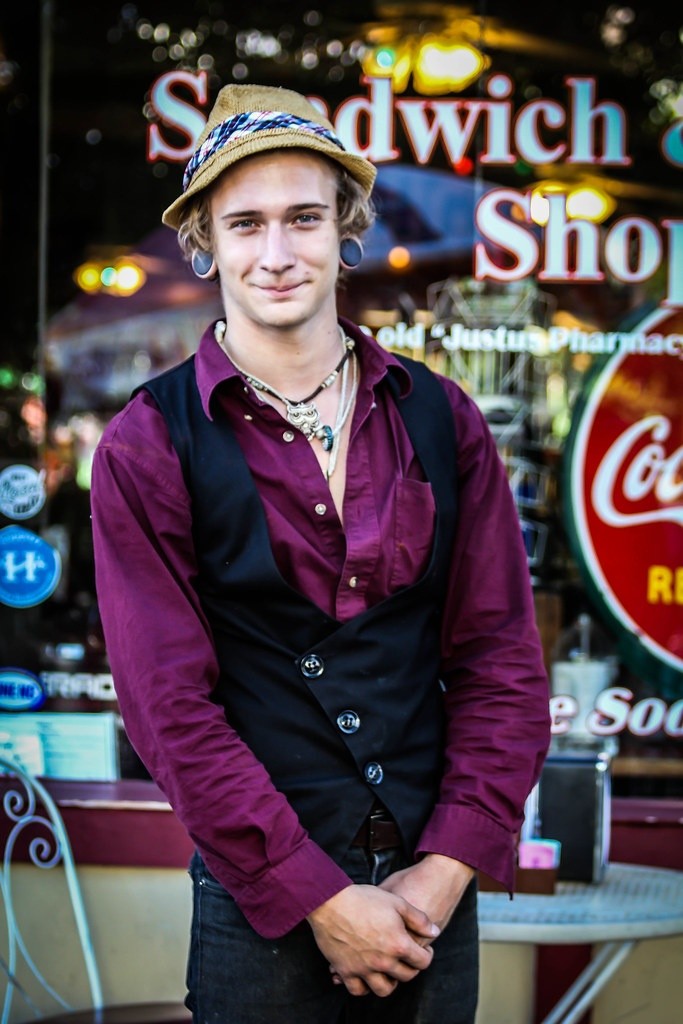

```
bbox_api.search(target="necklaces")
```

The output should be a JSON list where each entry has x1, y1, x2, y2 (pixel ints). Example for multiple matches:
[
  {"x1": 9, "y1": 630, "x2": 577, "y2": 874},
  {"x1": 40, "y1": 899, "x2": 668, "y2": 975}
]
[{"x1": 214, "y1": 319, "x2": 357, "y2": 487}]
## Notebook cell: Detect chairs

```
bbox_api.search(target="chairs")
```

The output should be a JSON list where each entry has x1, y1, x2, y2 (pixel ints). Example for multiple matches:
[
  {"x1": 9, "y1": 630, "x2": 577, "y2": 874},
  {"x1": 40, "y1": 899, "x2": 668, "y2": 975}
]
[{"x1": 0, "y1": 757, "x2": 192, "y2": 1024}]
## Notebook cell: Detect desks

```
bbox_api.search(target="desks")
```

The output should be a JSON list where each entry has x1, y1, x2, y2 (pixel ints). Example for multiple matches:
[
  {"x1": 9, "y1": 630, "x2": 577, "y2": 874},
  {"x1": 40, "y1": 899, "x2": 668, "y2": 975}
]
[{"x1": 477, "y1": 860, "x2": 683, "y2": 1024}]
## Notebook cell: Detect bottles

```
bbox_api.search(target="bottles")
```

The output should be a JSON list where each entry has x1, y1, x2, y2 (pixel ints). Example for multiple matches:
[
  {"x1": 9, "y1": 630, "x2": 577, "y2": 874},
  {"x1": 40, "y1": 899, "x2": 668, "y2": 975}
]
[{"x1": 539, "y1": 752, "x2": 613, "y2": 881}]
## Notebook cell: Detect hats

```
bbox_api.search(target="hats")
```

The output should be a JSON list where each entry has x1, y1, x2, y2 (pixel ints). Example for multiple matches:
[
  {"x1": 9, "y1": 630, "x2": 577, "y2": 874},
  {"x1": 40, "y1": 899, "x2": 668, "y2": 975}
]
[{"x1": 161, "y1": 83, "x2": 377, "y2": 230}]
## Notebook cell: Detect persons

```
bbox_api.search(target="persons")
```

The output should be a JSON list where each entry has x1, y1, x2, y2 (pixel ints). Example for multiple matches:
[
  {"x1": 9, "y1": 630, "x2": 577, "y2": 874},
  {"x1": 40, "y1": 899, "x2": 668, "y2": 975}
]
[{"x1": 91, "y1": 85, "x2": 550, "y2": 1024}]
